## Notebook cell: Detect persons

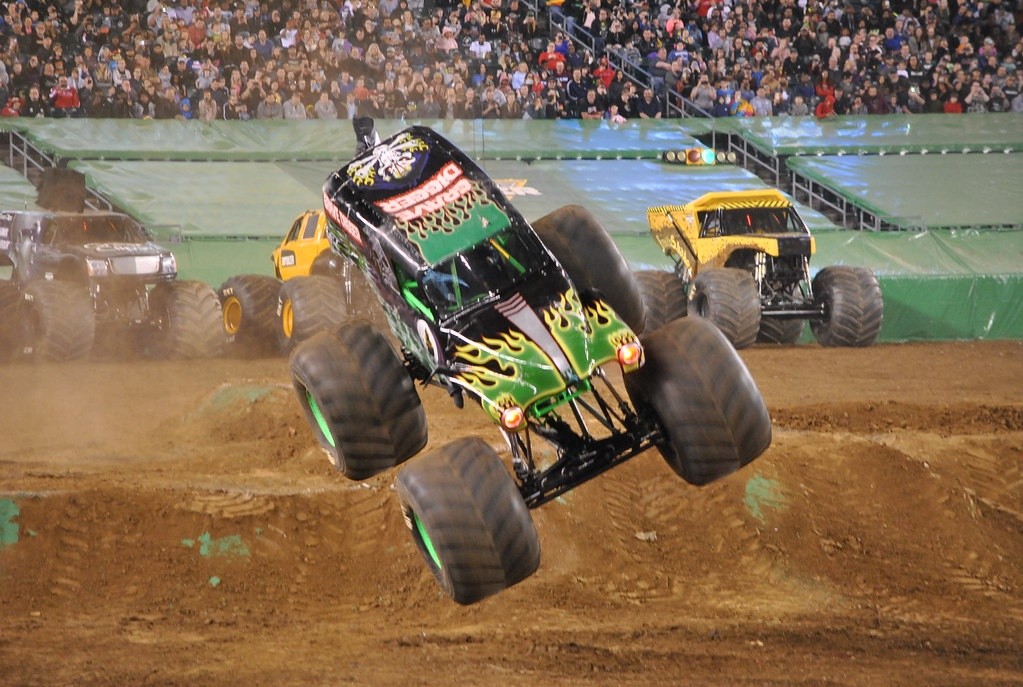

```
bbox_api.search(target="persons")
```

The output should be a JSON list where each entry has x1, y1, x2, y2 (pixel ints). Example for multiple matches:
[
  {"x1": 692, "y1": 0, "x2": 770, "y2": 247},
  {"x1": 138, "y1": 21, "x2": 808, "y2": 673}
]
[
  {"x1": 749, "y1": 86, "x2": 773, "y2": 117},
  {"x1": 815, "y1": 95, "x2": 838, "y2": 118},
  {"x1": 198, "y1": 88, "x2": 218, "y2": 119},
  {"x1": 636, "y1": 88, "x2": 661, "y2": 119},
  {"x1": 314, "y1": 90, "x2": 338, "y2": 119},
  {"x1": 963, "y1": 80, "x2": 990, "y2": 112},
  {"x1": 283, "y1": 91, "x2": 307, "y2": 119},
  {"x1": 0, "y1": 0, "x2": 1023, "y2": 119}
]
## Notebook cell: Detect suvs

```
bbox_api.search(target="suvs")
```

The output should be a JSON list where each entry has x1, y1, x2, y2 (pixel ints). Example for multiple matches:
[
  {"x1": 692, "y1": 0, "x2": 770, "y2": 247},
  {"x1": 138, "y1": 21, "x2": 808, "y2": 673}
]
[
  {"x1": 276, "y1": 126, "x2": 773, "y2": 607},
  {"x1": 626, "y1": 191, "x2": 884, "y2": 353},
  {"x1": 0, "y1": 208, "x2": 227, "y2": 370},
  {"x1": 214, "y1": 208, "x2": 372, "y2": 358}
]
